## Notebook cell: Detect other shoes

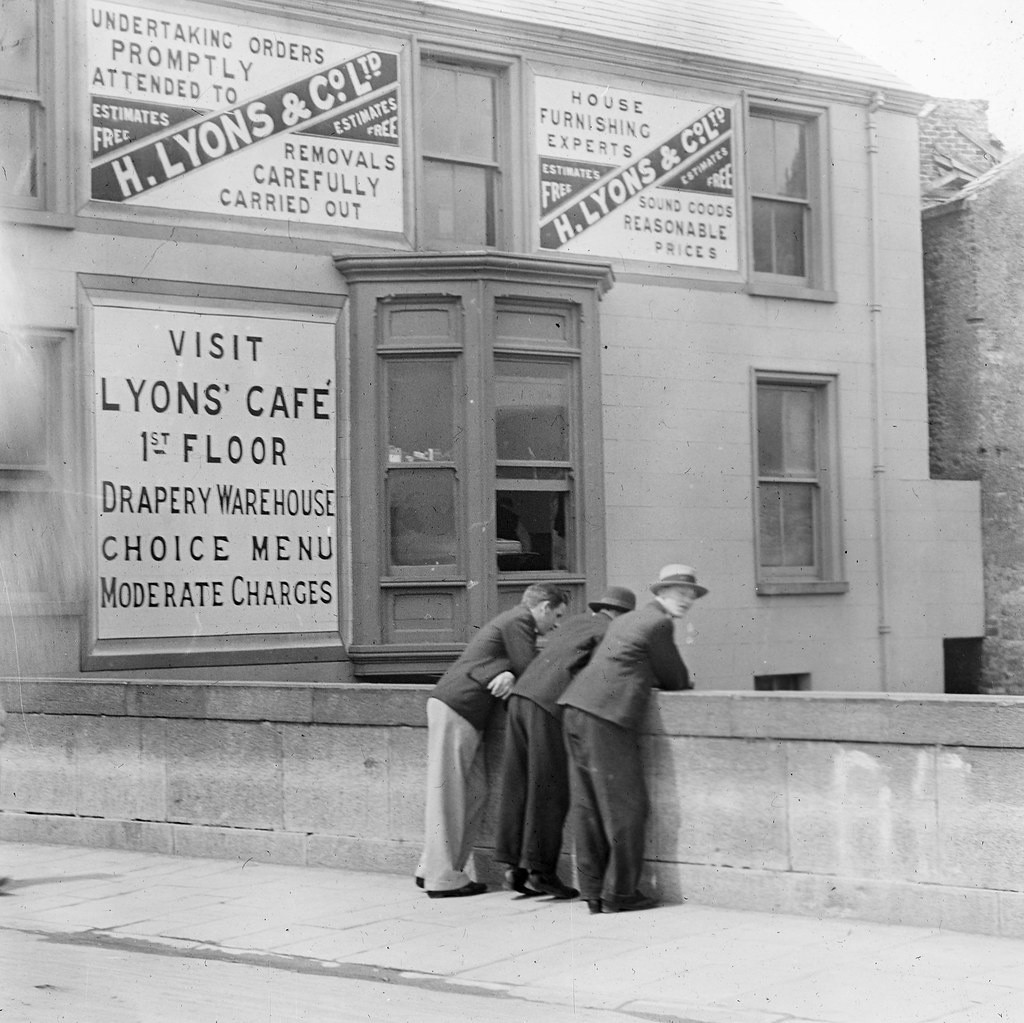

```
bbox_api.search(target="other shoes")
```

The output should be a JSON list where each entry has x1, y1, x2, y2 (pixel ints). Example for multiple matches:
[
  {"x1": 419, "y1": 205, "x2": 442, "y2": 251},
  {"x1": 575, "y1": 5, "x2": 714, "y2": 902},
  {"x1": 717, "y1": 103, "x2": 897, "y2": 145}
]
[
  {"x1": 587, "y1": 899, "x2": 602, "y2": 914},
  {"x1": 415, "y1": 877, "x2": 424, "y2": 888},
  {"x1": 601, "y1": 889, "x2": 660, "y2": 913},
  {"x1": 426, "y1": 881, "x2": 488, "y2": 899}
]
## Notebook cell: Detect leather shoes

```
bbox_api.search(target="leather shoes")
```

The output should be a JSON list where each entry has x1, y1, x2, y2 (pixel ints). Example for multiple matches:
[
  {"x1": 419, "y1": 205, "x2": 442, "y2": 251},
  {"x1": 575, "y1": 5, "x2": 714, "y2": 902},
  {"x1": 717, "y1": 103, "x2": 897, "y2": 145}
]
[
  {"x1": 524, "y1": 871, "x2": 580, "y2": 899},
  {"x1": 504, "y1": 867, "x2": 549, "y2": 896}
]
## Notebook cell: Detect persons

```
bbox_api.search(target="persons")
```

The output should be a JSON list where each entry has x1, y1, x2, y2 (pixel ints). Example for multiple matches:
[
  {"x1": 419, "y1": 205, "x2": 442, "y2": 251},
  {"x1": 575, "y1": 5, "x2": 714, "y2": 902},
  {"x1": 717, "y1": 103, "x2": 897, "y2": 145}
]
[
  {"x1": 414, "y1": 582, "x2": 568, "y2": 898},
  {"x1": 554, "y1": 565, "x2": 709, "y2": 914},
  {"x1": 492, "y1": 586, "x2": 635, "y2": 900}
]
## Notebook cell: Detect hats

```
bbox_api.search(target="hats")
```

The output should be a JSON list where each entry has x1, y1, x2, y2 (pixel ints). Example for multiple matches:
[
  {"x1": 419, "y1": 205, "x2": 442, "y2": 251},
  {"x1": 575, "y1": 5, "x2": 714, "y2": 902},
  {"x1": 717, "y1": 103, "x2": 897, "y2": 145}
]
[
  {"x1": 648, "y1": 563, "x2": 710, "y2": 598},
  {"x1": 587, "y1": 586, "x2": 636, "y2": 613}
]
